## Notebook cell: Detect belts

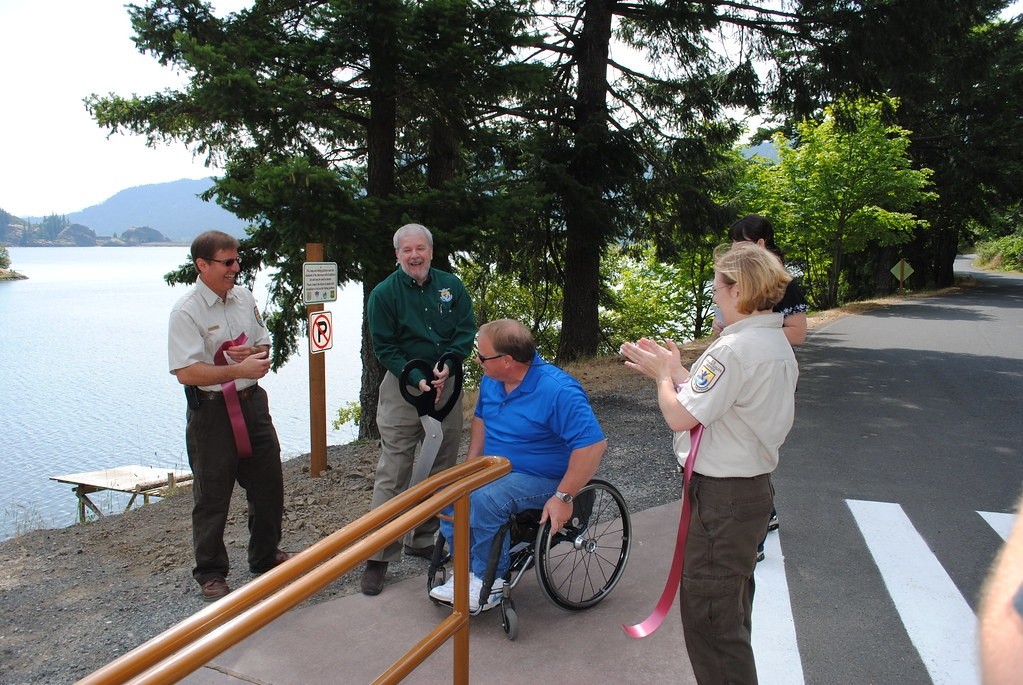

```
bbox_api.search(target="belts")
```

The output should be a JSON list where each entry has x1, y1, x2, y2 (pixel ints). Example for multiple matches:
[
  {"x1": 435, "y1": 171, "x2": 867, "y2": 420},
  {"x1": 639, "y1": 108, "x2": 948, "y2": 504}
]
[{"x1": 199, "y1": 382, "x2": 258, "y2": 401}]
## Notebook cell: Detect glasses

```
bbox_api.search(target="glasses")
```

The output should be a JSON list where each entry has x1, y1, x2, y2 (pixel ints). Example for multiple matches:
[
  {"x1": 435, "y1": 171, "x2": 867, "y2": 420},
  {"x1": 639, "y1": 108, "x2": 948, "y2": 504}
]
[
  {"x1": 476, "y1": 352, "x2": 504, "y2": 363},
  {"x1": 712, "y1": 281, "x2": 736, "y2": 294},
  {"x1": 204, "y1": 257, "x2": 243, "y2": 266}
]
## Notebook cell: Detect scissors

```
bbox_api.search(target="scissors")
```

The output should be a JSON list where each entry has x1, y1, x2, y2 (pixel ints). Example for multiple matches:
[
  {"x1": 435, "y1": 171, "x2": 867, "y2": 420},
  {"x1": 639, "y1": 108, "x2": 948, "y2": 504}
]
[{"x1": 396, "y1": 352, "x2": 463, "y2": 545}]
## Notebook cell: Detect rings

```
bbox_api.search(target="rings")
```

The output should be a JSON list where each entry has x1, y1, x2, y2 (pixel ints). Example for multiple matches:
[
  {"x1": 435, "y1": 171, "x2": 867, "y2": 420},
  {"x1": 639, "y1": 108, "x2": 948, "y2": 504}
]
[
  {"x1": 563, "y1": 521, "x2": 567, "y2": 524},
  {"x1": 240, "y1": 355, "x2": 242, "y2": 360}
]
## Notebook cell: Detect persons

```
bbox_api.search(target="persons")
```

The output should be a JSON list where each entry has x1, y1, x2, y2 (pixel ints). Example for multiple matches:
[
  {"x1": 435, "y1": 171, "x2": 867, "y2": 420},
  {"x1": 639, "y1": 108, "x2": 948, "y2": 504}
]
[
  {"x1": 168, "y1": 231, "x2": 293, "y2": 601},
  {"x1": 429, "y1": 319, "x2": 606, "y2": 612},
  {"x1": 360, "y1": 222, "x2": 477, "y2": 597},
  {"x1": 620, "y1": 241, "x2": 799, "y2": 685},
  {"x1": 978, "y1": 493, "x2": 1023, "y2": 685},
  {"x1": 713, "y1": 214, "x2": 808, "y2": 559}
]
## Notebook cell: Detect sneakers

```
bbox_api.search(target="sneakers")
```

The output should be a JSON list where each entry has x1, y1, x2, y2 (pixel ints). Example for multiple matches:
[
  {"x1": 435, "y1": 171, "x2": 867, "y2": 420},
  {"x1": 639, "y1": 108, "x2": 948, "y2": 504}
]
[
  {"x1": 756, "y1": 545, "x2": 765, "y2": 562},
  {"x1": 361, "y1": 560, "x2": 388, "y2": 595},
  {"x1": 766, "y1": 514, "x2": 780, "y2": 531},
  {"x1": 429, "y1": 572, "x2": 475, "y2": 602},
  {"x1": 201, "y1": 576, "x2": 230, "y2": 602},
  {"x1": 403, "y1": 544, "x2": 451, "y2": 563},
  {"x1": 249, "y1": 548, "x2": 301, "y2": 573},
  {"x1": 450, "y1": 577, "x2": 507, "y2": 612}
]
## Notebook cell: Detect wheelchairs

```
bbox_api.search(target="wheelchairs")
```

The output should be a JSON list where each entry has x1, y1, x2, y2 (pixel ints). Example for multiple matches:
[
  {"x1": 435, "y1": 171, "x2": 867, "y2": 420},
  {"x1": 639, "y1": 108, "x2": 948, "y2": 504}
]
[{"x1": 426, "y1": 476, "x2": 633, "y2": 640}]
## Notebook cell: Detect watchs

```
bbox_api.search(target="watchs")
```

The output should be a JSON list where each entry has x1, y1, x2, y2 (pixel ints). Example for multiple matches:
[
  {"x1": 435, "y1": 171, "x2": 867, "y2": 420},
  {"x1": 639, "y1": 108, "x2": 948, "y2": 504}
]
[{"x1": 555, "y1": 489, "x2": 574, "y2": 504}]
